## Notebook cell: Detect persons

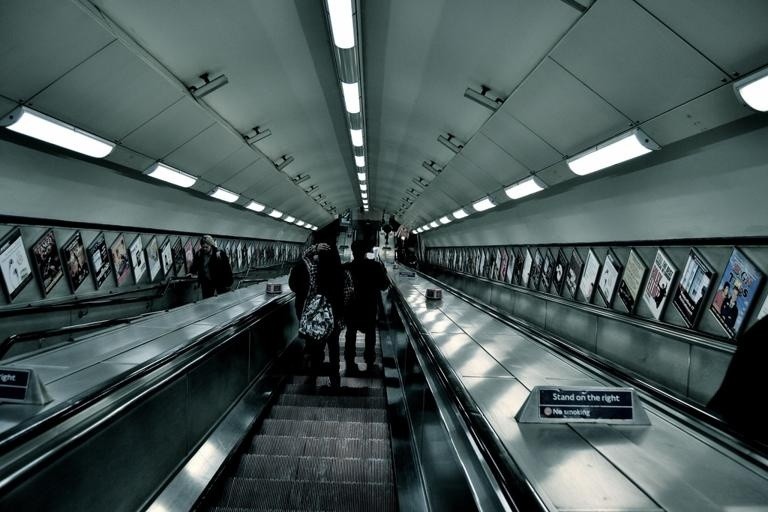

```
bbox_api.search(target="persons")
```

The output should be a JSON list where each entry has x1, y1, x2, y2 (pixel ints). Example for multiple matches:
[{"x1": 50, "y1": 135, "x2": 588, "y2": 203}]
[
  {"x1": 287, "y1": 230, "x2": 346, "y2": 394},
  {"x1": 189, "y1": 234, "x2": 234, "y2": 298},
  {"x1": 342, "y1": 239, "x2": 391, "y2": 377}
]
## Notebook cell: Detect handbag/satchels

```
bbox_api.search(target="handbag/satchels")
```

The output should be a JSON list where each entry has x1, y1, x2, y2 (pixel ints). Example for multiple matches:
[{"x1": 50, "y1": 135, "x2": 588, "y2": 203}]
[{"x1": 297, "y1": 291, "x2": 336, "y2": 345}]
